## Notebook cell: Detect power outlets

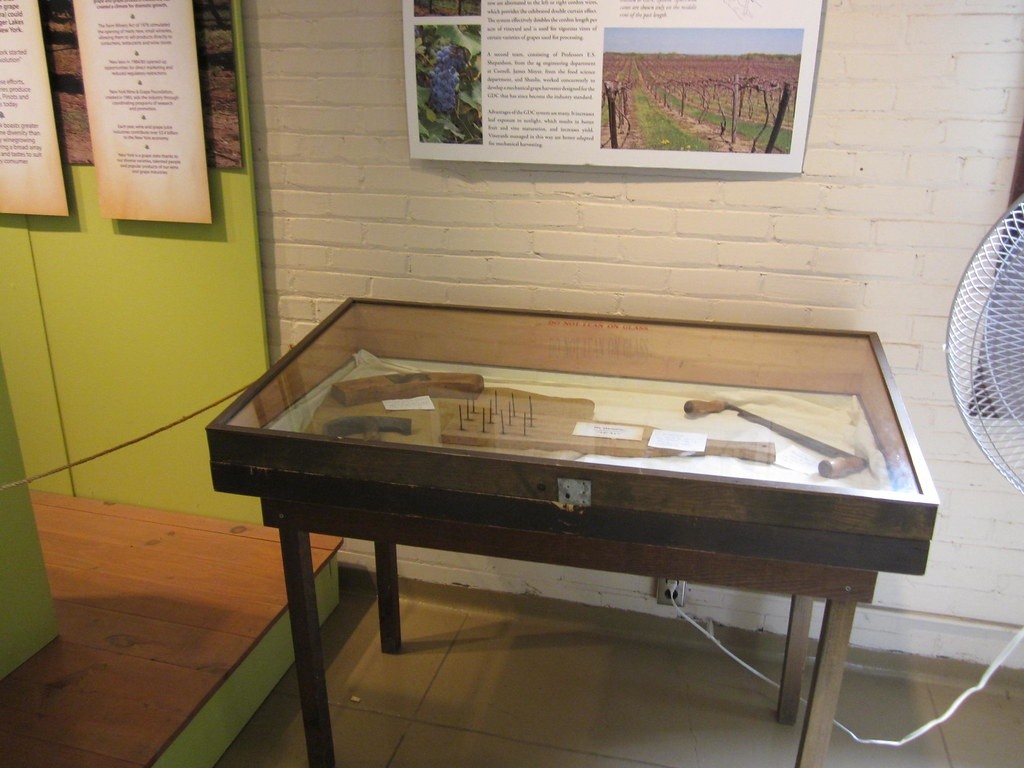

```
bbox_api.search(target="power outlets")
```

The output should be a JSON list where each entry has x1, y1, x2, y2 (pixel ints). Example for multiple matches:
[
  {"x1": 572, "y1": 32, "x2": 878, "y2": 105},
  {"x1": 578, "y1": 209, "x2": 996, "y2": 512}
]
[{"x1": 655, "y1": 577, "x2": 687, "y2": 608}]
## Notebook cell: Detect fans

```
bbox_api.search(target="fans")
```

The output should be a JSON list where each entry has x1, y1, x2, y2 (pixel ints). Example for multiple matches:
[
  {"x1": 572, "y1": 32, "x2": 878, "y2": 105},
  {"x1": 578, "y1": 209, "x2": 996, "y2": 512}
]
[{"x1": 943, "y1": 193, "x2": 1024, "y2": 498}]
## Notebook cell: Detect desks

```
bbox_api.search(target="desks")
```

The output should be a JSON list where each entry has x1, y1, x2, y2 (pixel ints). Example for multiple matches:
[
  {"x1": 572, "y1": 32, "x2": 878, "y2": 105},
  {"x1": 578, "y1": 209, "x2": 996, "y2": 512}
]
[{"x1": 260, "y1": 497, "x2": 879, "y2": 768}]
